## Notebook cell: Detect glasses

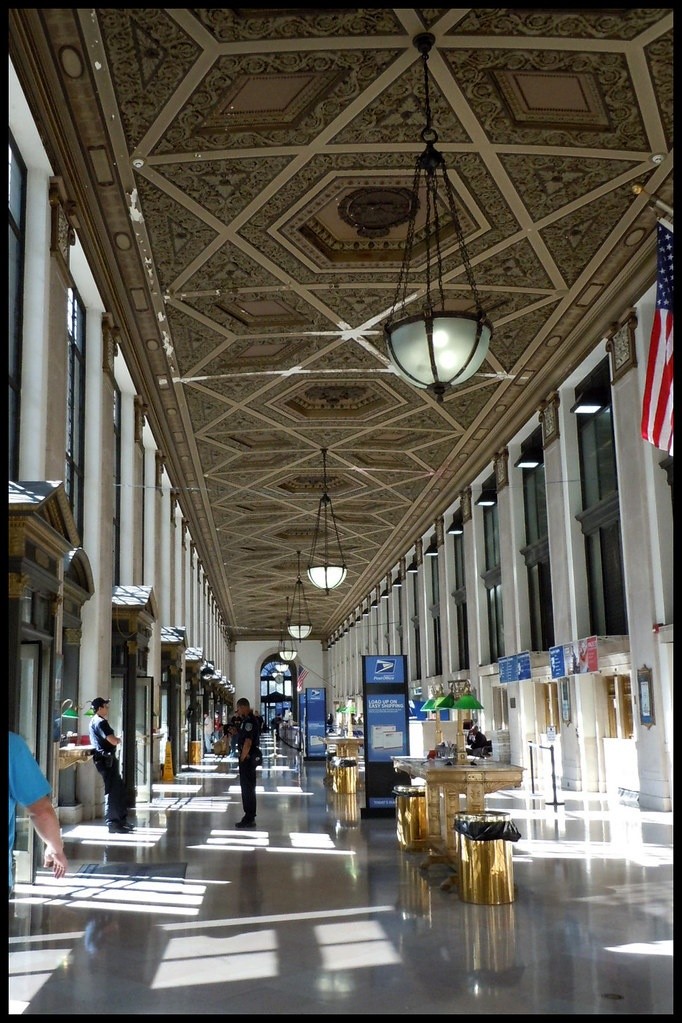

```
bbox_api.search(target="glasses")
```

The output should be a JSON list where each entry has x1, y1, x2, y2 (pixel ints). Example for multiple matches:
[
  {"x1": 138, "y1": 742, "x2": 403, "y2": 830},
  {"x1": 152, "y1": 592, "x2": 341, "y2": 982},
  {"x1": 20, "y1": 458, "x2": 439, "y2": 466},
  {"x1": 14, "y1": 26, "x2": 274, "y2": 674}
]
[{"x1": 103, "y1": 704, "x2": 109, "y2": 707}]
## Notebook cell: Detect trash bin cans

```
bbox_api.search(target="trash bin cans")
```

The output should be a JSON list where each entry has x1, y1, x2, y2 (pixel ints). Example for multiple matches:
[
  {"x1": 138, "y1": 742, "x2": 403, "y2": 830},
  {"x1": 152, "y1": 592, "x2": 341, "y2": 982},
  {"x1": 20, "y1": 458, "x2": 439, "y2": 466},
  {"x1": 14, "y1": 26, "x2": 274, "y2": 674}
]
[
  {"x1": 325, "y1": 750, "x2": 336, "y2": 775},
  {"x1": 189, "y1": 741, "x2": 201, "y2": 765},
  {"x1": 332, "y1": 756, "x2": 357, "y2": 793},
  {"x1": 454, "y1": 810, "x2": 515, "y2": 905},
  {"x1": 393, "y1": 785, "x2": 426, "y2": 845}
]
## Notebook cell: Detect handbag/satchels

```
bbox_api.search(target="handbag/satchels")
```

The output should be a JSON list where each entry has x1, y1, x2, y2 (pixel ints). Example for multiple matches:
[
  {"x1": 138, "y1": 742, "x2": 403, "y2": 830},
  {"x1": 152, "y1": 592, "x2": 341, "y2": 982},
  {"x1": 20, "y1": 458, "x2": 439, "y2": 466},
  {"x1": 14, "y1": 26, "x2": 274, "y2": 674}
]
[{"x1": 253, "y1": 747, "x2": 262, "y2": 766}]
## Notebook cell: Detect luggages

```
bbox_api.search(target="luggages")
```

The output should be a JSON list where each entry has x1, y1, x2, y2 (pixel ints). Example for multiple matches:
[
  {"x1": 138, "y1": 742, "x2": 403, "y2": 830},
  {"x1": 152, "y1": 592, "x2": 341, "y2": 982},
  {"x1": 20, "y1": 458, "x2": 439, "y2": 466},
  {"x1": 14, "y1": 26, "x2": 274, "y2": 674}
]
[{"x1": 214, "y1": 733, "x2": 230, "y2": 758}]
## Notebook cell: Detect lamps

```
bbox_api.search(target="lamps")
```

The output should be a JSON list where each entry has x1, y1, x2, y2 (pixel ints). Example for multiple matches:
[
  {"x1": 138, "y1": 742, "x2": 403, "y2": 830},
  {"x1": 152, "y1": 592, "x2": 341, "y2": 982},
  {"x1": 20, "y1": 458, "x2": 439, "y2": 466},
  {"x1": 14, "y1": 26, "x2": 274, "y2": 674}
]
[
  {"x1": 515, "y1": 444, "x2": 543, "y2": 470},
  {"x1": 445, "y1": 518, "x2": 464, "y2": 535},
  {"x1": 337, "y1": 701, "x2": 356, "y2": 735},
  {"x1": 380, "y1": 589, "x2": 389, "y2": 598},
  {"x1": 423, "y1": 545, "x2": 439, "y2": 556},
  {"x1": 474, "y1": 484, "x2": 497, "y2": 507},
  {"x1": 406, "y1": 560, "x2": 418, "y2": 573},
  {"x1": 271, "y1": 671, "x2": 283, "y2": 683},
  {"x1": 420, "y1": 683, "x2": 447, "y2": 759},
  {"x1": 82, "y1": 698, "x2": 96, "y2": 717},
  {"x1": 383, "y1": 34, "x2": 496, "y2": 404},
  {"x1": 436, "y1": 679, "x2": 484, "y2": 766},
  {"x1": 59, "y1": 696, "x2": 79, "y2": 720},
  {"x1": 279, "y1": 610, "x2": 298, "y2": 661},
  {"x1": 274, "y1": 651, "x2": 289, "y2": 672},
  {"x1": 327, "y1": 597, "x2": 378, "y2": 649},
  {"x1": 307, "y1": 446, "x2": 347, "y2": 596},
  {"x1": 568, "y1": 388, "x2": 603, "y2": 414},
  {"x1": 390, "y1": 577, "x2": 402, "y2": 587},
  {"x1": 287, "y1": 551, "x2": 313, "y2": 643}
]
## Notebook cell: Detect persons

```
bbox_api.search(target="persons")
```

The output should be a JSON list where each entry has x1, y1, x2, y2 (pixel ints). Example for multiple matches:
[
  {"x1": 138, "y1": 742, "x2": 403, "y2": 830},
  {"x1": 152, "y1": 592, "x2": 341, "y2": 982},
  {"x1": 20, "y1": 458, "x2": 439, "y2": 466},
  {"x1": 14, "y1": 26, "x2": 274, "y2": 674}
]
[
  {"x1": 9, "y1": 733, "x2": 68, "y2": 899},
  {"x1": 351, "y1": 712, "x2": 364, "y2": 737},
  {"x1": 204, "y1": 710, "x2": 282, "y2": 758},
  {"x1": 234, "y1": 698, "x2": 259, "y2": 828},
  {"x1": 89, "y1": 697, "x2": 134, "y2": 834},
  {"x1": 570, "y1": 645, "x2": 578, "y2": 674},
  {"x1": 466, "y1": 725, "x2": 486, "y2": 756},
  {"x1": 327, "y1": 713, "x2": 333, "y2": 733}
]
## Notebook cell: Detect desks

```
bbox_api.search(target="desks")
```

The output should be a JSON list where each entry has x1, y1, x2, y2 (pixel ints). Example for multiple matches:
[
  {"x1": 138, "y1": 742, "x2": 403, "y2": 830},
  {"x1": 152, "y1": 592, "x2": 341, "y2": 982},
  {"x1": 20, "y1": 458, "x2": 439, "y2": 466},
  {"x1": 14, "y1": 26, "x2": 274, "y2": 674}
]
[
  {"x1": 391, "y1": 756, "x2": 527, "y2": 891},
  {"x1": 319, "y1": 736, "x2": 364, "y2": 784}
]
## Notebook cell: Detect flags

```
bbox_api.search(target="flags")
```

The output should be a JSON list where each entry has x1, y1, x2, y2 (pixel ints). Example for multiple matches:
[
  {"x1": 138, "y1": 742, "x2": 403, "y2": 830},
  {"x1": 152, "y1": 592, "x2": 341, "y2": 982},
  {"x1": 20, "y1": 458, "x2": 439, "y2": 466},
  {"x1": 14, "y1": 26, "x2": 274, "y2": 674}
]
[
  {"x1": 639, "y1": 215, "x2": 674, "y2": 458},
  {"x1": 297, "y1": 665, "x2": 309, "y2": 691}
]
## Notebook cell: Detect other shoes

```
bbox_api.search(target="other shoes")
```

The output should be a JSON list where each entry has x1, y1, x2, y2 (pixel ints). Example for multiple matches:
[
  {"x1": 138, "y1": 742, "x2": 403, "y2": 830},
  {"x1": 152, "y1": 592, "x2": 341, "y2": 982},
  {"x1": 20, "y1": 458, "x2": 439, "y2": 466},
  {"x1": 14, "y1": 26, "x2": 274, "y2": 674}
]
[
  {"x1": 109, "y1": 826, "x2": 129, "y2": 833},
  {"x1": 125, "y1": 822, "x2": 135, "y2": 828},
  {"x1": 235, "y1": 820, "x2": 257, "y2": 829}
]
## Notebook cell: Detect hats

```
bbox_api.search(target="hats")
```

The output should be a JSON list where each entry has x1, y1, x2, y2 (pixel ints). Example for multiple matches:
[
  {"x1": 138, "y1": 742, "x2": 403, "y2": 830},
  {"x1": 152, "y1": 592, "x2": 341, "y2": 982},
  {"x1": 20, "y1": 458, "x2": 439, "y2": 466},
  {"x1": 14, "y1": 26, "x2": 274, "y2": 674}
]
[{"x1": 92, "y1": 697, "x2": 111, "y2": 712}]
